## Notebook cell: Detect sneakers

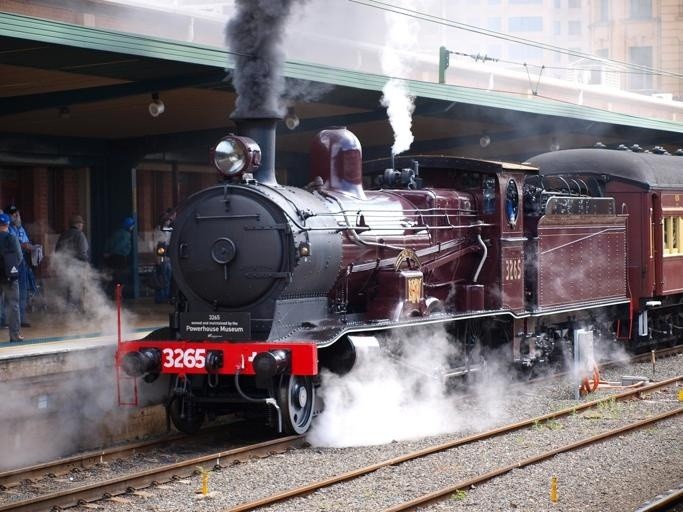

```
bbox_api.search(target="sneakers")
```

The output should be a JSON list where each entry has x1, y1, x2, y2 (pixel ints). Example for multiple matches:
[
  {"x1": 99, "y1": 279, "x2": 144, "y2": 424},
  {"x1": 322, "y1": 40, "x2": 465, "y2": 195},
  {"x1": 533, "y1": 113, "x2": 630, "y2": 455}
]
[
  {"x1": 10, "y1": 335, "x2": 24, "y2": 342},
  {"x1": 21, "y1": 321, "x2": 30, "y2": 327}
]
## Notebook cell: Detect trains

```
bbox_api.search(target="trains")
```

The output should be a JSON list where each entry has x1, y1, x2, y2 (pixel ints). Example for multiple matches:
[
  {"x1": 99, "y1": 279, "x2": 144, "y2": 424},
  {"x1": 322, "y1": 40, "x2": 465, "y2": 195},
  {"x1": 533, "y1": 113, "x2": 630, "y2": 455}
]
[{"x1": 108, "y1": 114, "x2": 681, "y2": 439}]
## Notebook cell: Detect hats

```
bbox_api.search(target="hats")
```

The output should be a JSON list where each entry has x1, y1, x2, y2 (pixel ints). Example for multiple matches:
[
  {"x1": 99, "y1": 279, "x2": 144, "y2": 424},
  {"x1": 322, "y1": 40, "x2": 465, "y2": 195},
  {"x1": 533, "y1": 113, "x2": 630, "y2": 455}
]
[
  {"x1": 69, "y1": 215, "x2": 85, "y2": 225},
  {"x1": 124, "y1": 217, "x2": 134, "y2": 229},
  {"x1": 1, "y1": 213, "x2": 10, "y2": 225}
]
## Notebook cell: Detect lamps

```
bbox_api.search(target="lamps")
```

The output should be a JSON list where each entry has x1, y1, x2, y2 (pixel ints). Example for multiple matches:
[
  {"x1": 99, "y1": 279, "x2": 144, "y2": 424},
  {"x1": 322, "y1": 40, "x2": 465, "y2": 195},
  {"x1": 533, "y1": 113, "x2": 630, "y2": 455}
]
[
  {"x1": 285, "y1": 103, "x2": 299, "y2": 132},
  {"x1": 148, "y1": 91, "x2": 165, "y2": 117}
]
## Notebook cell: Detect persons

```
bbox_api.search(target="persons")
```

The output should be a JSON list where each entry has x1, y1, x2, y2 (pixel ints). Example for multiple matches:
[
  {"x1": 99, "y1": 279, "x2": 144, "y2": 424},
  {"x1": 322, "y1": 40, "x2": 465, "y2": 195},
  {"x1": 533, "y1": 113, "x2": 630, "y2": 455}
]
[
  {"x1": 55, "y1": 215, "x2": 89, "y2": 318},
  {"x1": 0, "y1": 205, "x2": 40, "y2": 343},
  {"x1": 102, "y1": 217, "x2": 136, "y2": 306}
]
